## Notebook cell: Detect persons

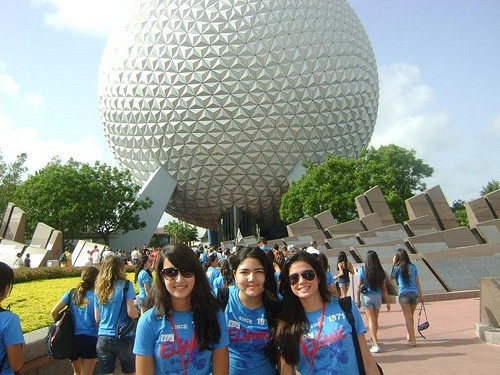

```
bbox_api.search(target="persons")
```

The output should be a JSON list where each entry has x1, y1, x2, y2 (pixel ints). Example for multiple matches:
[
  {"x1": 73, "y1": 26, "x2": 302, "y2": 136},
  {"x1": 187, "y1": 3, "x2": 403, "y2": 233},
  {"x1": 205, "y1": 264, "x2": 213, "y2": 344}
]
[
  {"x1": 14, "y1": 237, "x2": 391, "y2": 375},
  {"x1": 0, "y1": 261, "x2": 26, "y2": 375},
  {"x1": 390, "y1": 248, "x2": 424, "y2": 347}
]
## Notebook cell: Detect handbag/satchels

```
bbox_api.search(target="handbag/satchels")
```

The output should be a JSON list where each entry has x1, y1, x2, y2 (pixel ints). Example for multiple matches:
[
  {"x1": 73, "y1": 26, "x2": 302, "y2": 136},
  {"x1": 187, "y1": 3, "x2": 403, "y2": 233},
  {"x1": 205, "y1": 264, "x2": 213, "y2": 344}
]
[
  {"x1": 115, "y1": 280, "x2": 137, "y2": 346},
  {"x1": 359, "y1": 265, "x2": 369, "y2": 294},
  {"x1": 418, "y1": 302, "x2": 429, "y2": 332},
  {"x1": 338, "y1": 294, "x2": 383, "y2": 375}
]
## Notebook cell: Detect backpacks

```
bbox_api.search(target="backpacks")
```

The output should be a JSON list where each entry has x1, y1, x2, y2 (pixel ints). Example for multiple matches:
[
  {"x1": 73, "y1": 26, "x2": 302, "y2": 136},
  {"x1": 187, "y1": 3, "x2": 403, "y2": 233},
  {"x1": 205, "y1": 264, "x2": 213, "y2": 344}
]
[
  {"x1": 46, "y1": 287, "x2": 79, "y2": 362},
  {"x1": 59, "y1": 252, "x2": 71, "y2": 264}
]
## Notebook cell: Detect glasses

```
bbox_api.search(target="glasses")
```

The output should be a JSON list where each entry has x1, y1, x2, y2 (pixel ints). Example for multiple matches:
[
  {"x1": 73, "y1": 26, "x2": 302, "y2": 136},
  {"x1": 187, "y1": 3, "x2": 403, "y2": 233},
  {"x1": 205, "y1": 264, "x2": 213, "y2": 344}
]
[
  {"x1": 161, "y1": 267, "x2": 195, "y2": 281},
  {"x1": 286, "y1": 270, "x2": 315, "y2": 285}
]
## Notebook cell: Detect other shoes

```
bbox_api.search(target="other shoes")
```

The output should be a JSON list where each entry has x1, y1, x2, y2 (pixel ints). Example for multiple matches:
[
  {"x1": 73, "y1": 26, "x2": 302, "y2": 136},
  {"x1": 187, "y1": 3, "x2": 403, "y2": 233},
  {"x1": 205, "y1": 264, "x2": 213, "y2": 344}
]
[{"x1": 407, "y1": 335, "x2": 416, "y2": 347}]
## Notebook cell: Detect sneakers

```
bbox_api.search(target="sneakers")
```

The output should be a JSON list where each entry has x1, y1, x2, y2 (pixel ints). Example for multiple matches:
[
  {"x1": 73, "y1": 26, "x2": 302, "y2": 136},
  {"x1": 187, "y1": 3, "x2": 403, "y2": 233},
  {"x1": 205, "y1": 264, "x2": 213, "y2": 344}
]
[{"x1": 369, "y1": 338, "x2": 381, "y2": 352}]
